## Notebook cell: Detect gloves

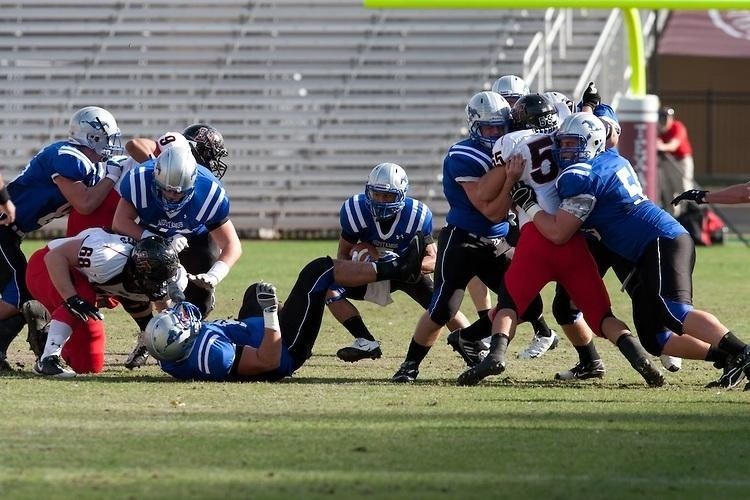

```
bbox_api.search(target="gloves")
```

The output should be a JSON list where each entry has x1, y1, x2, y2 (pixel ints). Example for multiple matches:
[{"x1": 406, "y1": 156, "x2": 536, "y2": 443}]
[
  {"x1": 187, "y1": 273, "x2": 216, "y2": 290},
  {"x1": 671, "y1": 189, "x2": 710, "y2": 206},
  {"x1": 509, "y1": 181, "x2": 539, "y2": 213},
  {"x1": 166, "y1": 233, "x2": 189, "y2": 253},
  {"x1": 105, "y1": 159, "x2": 124, "y2": 182},
  {"x1": 62, "y1": 295, "x2": 104, "y2": 323},
  {"x1": 255, "y1": 280, "x2": 279, "y2": 312}
]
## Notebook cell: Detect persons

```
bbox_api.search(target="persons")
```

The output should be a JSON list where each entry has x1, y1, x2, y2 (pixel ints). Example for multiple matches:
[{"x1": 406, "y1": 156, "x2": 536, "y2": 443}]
[
  {"x1": 455, "y1": 82, "x2": 669, "y2": 388},
  {"x1": 26, "y1": 228, "x2": 178, "y2": 377},
  {"x1": 392, "y1": 92, "x2": 544, "y2": 383},
  {"x1": 325, "y1": 161, "x2": 506, "y2": 376},
  {"x1": 510, "y1": 110, "x2": 750, "y2": 390},
  {"x1": 546, "y1": 91, "x2": 680, "y2": 379},
  {"x1": 113, "y1": 123, "x2": 228, "y2": 310},
  {"x1": 113, "y1": 146, "x2": 242, "y2": 370},
  {"x1": 142, "y1": 228, "x2": 426, "y2": 382},
  {"x1": 485, "y1": 77, "x2": 560, "y2": 361},
  {"x1": 2, "y1": 107, "x2": 127, "y2": 376}
]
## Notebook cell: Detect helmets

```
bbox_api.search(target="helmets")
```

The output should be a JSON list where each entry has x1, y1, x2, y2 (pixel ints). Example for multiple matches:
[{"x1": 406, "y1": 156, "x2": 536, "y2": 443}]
[
  {"x1": 364, "y1": 162, "x2": 409, "y2": 222},
  {"x1": 151, "y1": 124, "x2": 228, "y2": 212},
  {"x1": 130, "y1": 236, "x2": 178, "y2": 289},
  {"x1": 69, "y1": 107, "x2": 124, "y2": 163},
  {"x1": 464, "y1": 75, "x2": 608, "y2": 169},
  {"x1": 144, "y1": 301, "x2": 203, "y2": 359}
]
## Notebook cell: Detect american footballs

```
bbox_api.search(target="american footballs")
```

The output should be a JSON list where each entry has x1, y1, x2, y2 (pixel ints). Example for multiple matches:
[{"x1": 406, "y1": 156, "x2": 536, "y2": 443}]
[{"x1": 348, "y1": 242, "x2": 379, "y2": 261}]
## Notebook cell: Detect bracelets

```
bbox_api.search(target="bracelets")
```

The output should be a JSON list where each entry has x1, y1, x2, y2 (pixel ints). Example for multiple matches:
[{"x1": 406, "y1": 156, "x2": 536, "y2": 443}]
[{"x1": 262, "y1": 311, "x2": 279, "y2": 332}]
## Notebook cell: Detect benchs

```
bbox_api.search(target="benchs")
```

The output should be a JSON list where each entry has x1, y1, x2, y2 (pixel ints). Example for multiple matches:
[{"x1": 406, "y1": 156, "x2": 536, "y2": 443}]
[{"x1": 0, "y1": 0, "x2": 652, "y2": 239}]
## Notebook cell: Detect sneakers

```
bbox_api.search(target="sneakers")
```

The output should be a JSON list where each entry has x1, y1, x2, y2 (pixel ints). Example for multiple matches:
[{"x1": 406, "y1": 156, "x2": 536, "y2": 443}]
[
  {"x1": 0, "y1": 300, "x2": 77, "y2": 379},
  {"x1": 635, "y1": 353, "x2": 684, "y2": 388},
  {"x1": 125, "y1": 331, "x2": 150, "y2": 370},
  {"x1": 392, "y1": 361, "x2": 419, "y2": 384},
  {"x1": 337, "y1": 337, "x2": 382, "y2": 363},
  {"x1": 521, "y1": 328, "x2": 559, "y2": 359},
  {"x1": 398, "y1": 230, "x2": 425, "y2": 283},
  {"x1": 447, "y1": 328, "x2": 505, "y2": 385},
  {"x1": 705, "y1": 344, "x2": 750, "y2": 391},
  {"x1": 555, "y1": 359, "x2": 606, "y2": 380}
]
